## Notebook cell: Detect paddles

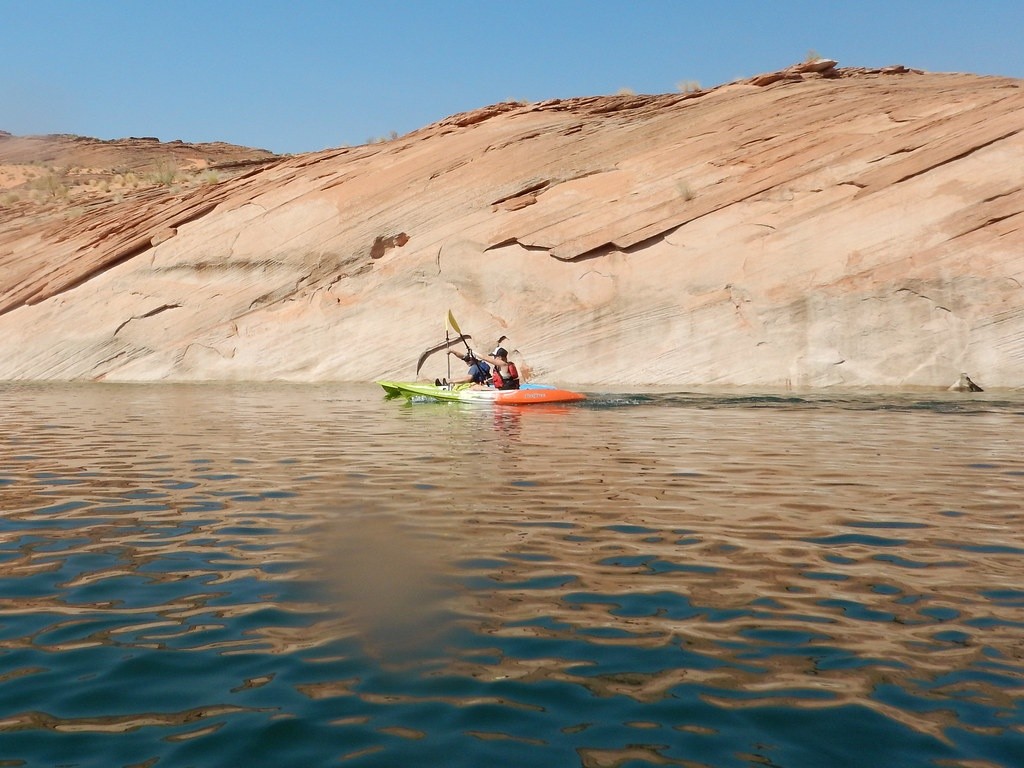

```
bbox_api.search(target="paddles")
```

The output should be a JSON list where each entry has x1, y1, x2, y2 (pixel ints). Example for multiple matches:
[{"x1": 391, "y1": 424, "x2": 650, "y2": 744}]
[
  {"x1": 444, "y1": 311, "x2": 451, "y2": 391},
  {"x1": 447, "y1": 308, "x2": 493, "y2": 389}
]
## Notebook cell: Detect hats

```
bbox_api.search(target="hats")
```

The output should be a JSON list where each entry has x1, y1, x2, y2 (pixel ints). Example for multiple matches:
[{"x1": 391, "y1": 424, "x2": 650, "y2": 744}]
[
  {"x1": 462, "y1": 353, "x2": 476, "y2": 360},
  {"x1": 488, "y1": 346, "x2": 508, "y2": 356}
]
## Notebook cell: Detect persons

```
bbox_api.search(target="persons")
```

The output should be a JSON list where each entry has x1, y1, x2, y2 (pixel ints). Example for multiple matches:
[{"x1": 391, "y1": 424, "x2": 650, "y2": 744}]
[
  {"x1": 434, "y1": 349, "x2": 492, "y2": 387},
  {"x1": 466, "y1": 346, "x2": 520, "y2": 392}
]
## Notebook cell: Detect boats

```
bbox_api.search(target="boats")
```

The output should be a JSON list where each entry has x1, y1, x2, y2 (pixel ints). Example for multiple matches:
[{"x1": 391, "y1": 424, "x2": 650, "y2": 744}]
[{"x1": 376, "y1": 380, "x2": 588, "y2": 405}]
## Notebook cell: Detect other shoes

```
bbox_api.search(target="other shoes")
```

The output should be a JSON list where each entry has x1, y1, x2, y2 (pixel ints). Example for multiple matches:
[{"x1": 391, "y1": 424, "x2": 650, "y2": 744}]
[{"x1": 435, "y1": 378, "x2": 448, "y2": 386}]
